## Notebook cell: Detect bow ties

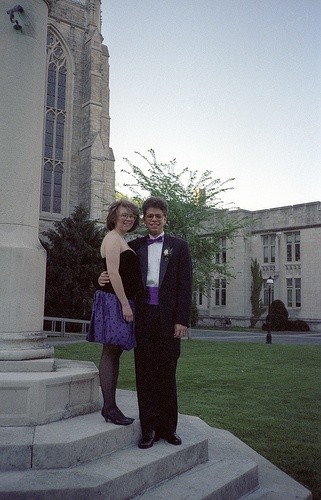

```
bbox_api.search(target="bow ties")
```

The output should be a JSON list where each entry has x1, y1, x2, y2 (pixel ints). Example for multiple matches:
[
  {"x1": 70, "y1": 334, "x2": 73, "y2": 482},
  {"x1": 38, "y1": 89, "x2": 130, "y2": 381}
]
[{"x1": 146, "y1": 236, "x2": 162, "y2": 245}]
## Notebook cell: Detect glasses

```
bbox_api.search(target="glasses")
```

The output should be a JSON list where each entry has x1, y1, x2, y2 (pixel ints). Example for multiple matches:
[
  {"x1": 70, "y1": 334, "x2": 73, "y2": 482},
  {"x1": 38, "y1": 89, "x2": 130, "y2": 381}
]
[
  {"x1": 145, "y1": 215, "x2": 163, "y2": 220},
  {"x1": 118, "y1": 214, "x2": 137, "y2": 221}
]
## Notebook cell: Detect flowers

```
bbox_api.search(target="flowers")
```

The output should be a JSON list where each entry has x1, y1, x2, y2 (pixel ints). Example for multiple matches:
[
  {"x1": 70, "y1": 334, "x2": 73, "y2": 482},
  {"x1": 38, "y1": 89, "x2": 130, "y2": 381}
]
[{"x1": 163, "y1": 247, "x2": 174, "y2": 262}]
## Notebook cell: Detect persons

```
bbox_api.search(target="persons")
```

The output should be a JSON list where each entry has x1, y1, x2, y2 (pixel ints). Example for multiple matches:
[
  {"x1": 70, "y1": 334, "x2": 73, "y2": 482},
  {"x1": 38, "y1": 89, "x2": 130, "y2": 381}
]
[
  {"x1": 84, "y1": 199, "x2": 142, "y2": 425},
  {"x1": 93, "y1": 196, "x2": 193, "y2": 449}
]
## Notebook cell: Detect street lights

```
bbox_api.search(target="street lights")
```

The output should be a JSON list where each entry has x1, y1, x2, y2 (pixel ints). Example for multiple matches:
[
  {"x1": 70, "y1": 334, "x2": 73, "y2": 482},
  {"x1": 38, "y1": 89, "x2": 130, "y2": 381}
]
[{"x1": 265, "y1": 276, "x2": 274, "y2": 344}]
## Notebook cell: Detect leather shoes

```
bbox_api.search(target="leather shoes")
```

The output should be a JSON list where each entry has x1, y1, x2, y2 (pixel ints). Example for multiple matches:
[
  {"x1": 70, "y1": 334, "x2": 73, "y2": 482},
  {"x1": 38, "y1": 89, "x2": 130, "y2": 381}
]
[
  {"x1": 138, "y1": 430, "x2": 159, "y2": 449},
  {"x1": 159, "y1": 432, "x2": 181, "y2": 445}
]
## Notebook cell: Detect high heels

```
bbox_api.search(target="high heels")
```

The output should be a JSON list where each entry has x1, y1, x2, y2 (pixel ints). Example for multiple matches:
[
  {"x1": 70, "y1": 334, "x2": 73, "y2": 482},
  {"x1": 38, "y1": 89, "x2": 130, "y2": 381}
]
[
  {"x1": 100, "y1": 406, "x2": 132, "y2": 425},
  {"x1": 115, "y1": 406, "x2": 134, "y2": 421}
]
